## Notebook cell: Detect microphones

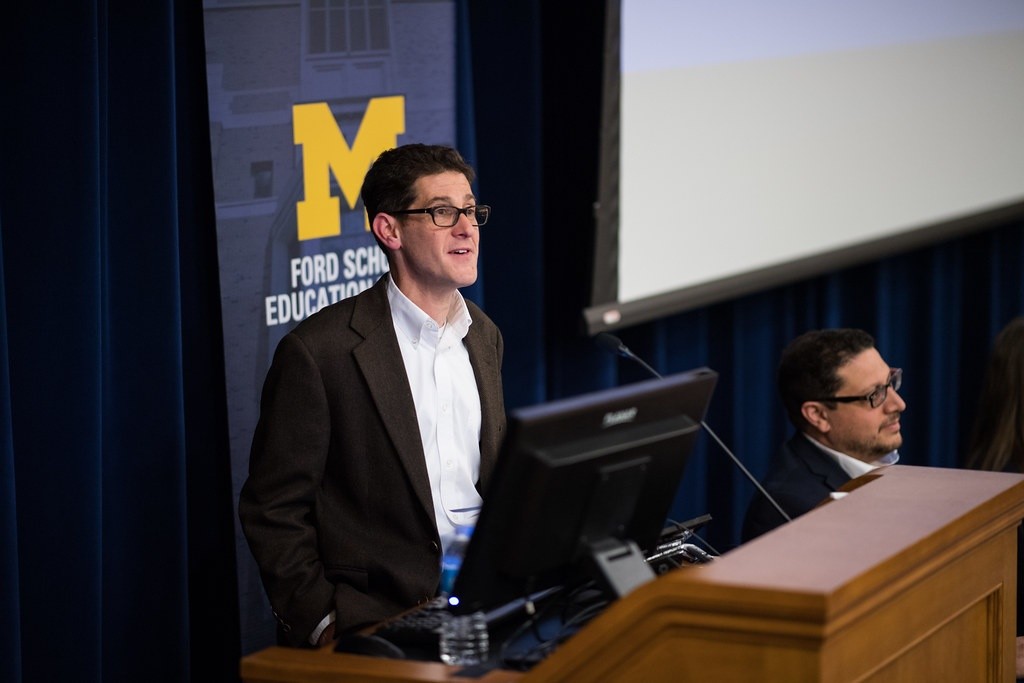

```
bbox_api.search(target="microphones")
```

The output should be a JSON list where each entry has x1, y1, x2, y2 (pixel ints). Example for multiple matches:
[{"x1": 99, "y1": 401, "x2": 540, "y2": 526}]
[{"x1": 597, "y1": 332, "x2": 792, "y2": 521}]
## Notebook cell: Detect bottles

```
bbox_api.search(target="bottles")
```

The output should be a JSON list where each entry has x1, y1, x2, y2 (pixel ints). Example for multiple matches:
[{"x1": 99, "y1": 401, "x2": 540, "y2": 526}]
[{"x1": 439, "y1": 524, "x2": 490, "y2": 667}]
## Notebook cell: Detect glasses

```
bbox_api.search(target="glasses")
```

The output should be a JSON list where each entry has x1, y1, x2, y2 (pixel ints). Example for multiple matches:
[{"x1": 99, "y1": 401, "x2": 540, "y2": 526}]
[
  {"x1": 803, "y1": 367, "x2": 903, "y2": 409},
  {"x1": 391, "y1": 206, "x2": 492, "y2": 229}
]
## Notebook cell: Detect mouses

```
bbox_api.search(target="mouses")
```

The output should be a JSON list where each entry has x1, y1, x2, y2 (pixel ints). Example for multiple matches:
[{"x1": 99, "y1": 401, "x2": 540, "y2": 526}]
[{"x1": 333, "y1": 636, "x2": 404, "y2": 658}]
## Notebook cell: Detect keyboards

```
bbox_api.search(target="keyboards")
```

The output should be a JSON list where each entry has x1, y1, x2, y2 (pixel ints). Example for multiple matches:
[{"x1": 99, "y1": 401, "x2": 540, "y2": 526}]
[{"x1": 375, "y1": 594, "x2": 447, "y2": 662}]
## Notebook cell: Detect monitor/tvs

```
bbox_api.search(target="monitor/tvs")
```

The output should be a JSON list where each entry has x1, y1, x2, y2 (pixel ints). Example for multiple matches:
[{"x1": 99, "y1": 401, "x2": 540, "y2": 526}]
[{"x1": 446, "y1": 395, "x2": 694, "y2": 619}]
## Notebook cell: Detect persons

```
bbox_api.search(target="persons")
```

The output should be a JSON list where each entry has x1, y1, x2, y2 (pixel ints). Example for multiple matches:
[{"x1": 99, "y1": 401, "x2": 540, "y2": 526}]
[
  {"x1": 741, "y1": 329, "x2": 907, "y2": 542},
  {"x1": 964, "y1": 315, "x2": 1023, "y2": 683},
  {"x1": 237, "y1": 143, "x2": 507, "y2": 650}
]
